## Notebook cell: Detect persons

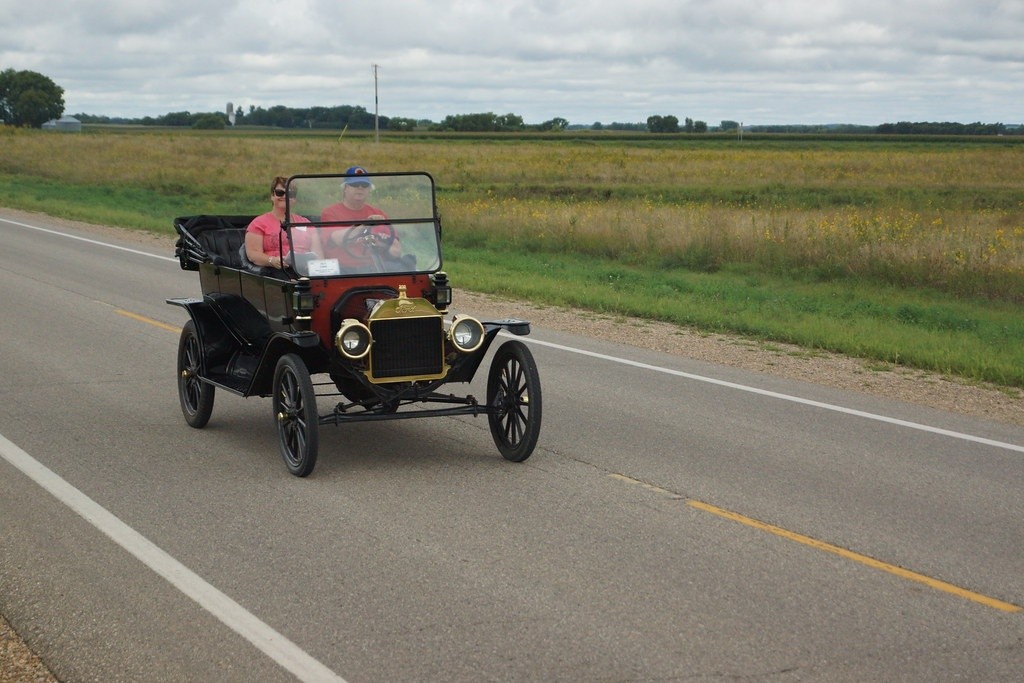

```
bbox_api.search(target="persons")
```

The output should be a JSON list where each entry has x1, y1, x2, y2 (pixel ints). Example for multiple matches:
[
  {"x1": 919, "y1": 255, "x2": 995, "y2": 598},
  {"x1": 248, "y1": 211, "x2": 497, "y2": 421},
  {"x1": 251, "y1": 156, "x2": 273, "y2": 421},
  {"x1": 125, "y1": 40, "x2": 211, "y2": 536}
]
[
  {"x1": 245, "y1": 176, "x2": 323, "y2": 277},
  {"x1": 322, "y1": 165, "x2": 413, "y2": 273}
]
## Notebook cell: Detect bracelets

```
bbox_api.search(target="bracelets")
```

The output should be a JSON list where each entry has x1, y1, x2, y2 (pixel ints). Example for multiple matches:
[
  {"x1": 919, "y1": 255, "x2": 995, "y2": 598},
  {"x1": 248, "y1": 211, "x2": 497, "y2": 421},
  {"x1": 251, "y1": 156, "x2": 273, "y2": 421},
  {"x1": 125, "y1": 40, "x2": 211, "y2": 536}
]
[{"x1": 268, "y1": 257, "x2": 272, "y2": 265}]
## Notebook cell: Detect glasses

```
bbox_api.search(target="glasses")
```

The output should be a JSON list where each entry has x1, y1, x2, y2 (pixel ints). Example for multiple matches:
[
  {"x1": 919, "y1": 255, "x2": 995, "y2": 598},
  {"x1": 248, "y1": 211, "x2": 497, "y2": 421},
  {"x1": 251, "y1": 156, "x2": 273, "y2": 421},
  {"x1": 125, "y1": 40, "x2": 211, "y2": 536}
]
[
  {"x1": 349, "y1": 182, "x2": 370, "y2": 188},
  {"x1": 273, "y1": 189, "x2": 296, "y2": 199}
]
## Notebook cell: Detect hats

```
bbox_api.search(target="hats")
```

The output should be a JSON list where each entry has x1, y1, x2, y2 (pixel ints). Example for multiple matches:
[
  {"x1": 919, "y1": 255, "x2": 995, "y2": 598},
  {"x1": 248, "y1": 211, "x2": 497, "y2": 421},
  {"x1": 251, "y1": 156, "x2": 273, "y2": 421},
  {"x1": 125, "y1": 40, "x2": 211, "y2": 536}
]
[{"x1": 345, "y1": 167, "x2": 371, "y2": 185}]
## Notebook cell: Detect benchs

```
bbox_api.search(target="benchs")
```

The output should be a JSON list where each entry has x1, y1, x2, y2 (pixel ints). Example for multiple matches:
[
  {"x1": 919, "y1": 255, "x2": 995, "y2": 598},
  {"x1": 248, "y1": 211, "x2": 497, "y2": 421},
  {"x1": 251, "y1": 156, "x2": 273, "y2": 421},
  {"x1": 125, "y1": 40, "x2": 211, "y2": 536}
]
[{"x1": 198, "y1": 228, "x2": 267, "y2": 275}]
